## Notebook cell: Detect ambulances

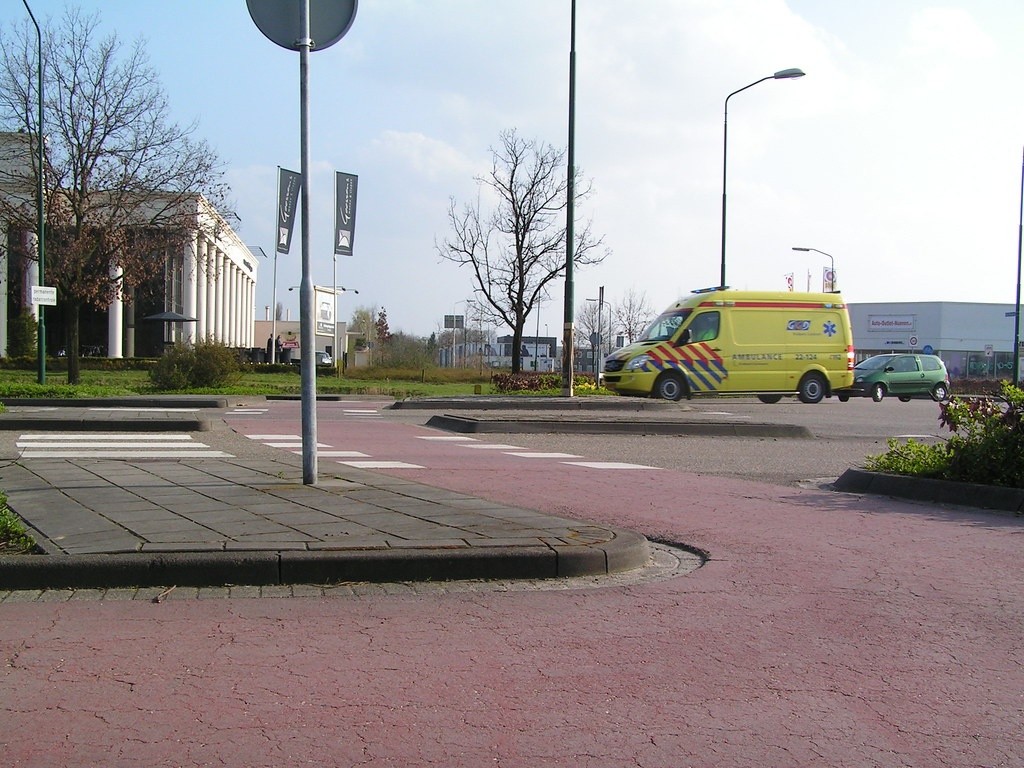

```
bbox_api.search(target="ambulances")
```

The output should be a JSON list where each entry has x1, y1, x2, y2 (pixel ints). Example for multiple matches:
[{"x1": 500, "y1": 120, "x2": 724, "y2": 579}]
[{"x1": 602, "y1": 285, "x2": 854, "y2": 399}]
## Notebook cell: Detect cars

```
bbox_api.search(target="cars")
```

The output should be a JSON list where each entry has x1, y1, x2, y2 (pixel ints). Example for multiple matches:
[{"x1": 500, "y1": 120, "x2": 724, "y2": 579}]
[
  {"x1": 836, "y1": 354, "x2": 950, "y2": 402},
  {"x1": 316, "y1": 351, "x2": 332, "y2": 368}
]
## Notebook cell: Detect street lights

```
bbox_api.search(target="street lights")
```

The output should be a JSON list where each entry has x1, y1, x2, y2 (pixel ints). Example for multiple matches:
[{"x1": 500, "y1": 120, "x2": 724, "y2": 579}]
[
  {"x1": 792, "y1": 247, "x2": 834, "y2": 293},
  {"x1": 720, "y1": 67, "x2": 808, "y2": 287}
]
